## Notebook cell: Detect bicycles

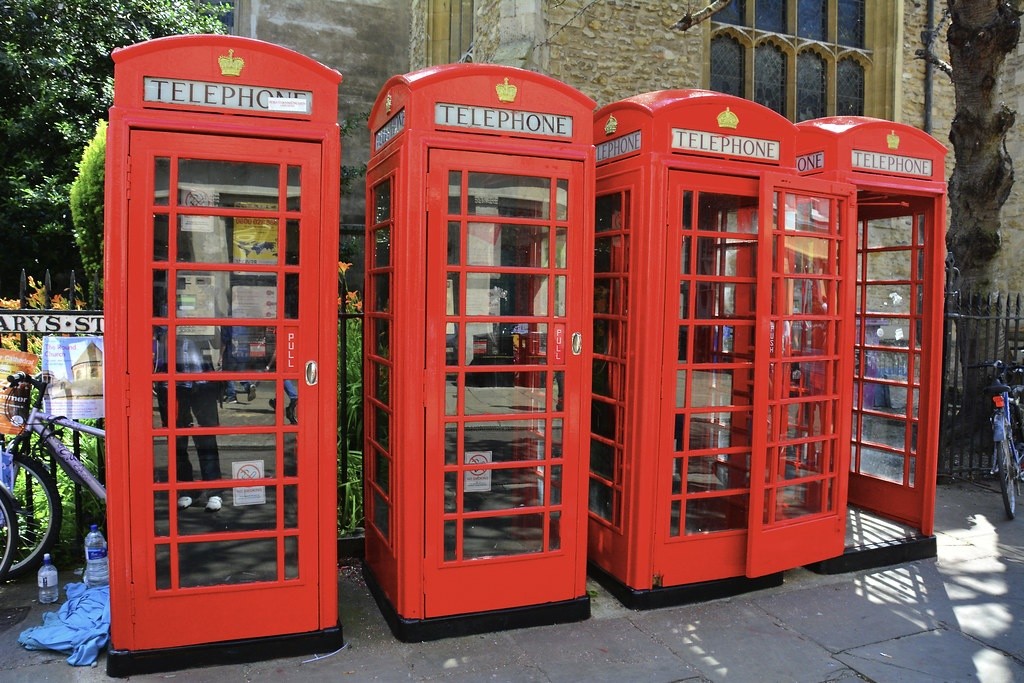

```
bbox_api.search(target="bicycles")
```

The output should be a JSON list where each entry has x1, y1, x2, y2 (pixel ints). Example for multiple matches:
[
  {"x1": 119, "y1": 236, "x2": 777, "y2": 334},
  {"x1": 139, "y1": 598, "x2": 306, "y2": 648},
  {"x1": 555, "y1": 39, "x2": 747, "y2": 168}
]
[
  {"x1": 0, "y1": 367, "x2": 107, "y2": 584},
  {"x1": 964, "y1": 356, "x2": 1024, "y2": 522}
]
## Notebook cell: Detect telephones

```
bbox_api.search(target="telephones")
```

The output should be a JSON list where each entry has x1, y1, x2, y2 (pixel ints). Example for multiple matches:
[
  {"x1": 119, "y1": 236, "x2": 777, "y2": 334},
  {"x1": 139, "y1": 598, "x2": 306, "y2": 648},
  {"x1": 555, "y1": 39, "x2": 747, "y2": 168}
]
[{"x1": 152, "y1": 270, "x2": 228, "y2": 366}]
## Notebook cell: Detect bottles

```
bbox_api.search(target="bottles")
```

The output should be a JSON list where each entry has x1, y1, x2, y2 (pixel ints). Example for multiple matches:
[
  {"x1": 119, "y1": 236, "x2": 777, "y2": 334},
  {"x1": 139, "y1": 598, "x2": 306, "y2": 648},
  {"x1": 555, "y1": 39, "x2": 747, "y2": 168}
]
[
  {"x1": 84, "y1": 525, "x2": 109, "y2": 588},
  {"x1": 38, "y1": 554, "x2": 59, "y2": 603}
]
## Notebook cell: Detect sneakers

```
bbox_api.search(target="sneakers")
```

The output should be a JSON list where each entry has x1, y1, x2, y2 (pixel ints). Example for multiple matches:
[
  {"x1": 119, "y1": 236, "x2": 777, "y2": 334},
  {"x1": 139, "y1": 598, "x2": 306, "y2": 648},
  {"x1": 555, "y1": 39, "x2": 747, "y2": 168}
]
[
  {"x1": 248, "y1": 384, "x2": 256, "y2": 401},
  {"x1": 178, "y1": 497, "x2": 192, "y2": 508},
  {"x1": 223, "y1": 396, "x2": 238, "y2": 403},
  {"x1": 205, "y1": 496, "x2": 222, "y2": 512}
]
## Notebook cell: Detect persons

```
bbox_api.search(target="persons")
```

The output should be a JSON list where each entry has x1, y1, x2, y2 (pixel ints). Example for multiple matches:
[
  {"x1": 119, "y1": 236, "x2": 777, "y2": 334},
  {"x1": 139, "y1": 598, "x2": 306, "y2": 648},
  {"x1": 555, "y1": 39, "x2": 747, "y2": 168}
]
[{"x1": 152, "y1": 288, "x2": 297, "y2": 511}]
